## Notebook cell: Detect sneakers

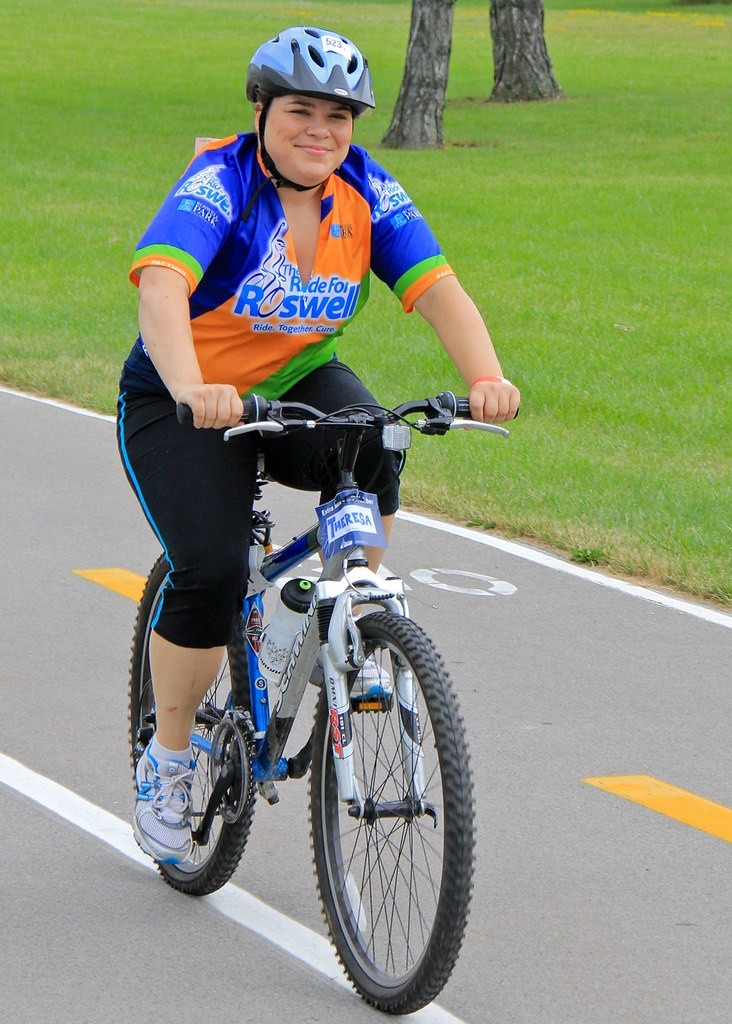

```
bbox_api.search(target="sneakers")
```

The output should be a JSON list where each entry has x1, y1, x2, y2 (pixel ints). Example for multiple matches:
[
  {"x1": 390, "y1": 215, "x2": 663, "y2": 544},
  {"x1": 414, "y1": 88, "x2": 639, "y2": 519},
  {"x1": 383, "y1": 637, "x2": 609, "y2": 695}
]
[
  {"x1": 131, "y1": 736, "x2": 198, "y2": 863},
  {"x1": 317, "y1": 640, "x2": 394, "y2": 702}
]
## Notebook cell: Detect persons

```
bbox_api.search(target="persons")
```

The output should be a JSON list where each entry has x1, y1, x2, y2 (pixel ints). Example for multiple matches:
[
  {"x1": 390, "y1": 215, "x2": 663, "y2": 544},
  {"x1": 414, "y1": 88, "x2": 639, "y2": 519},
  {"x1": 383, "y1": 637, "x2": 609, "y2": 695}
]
[{"x1": 113, "y1": 46, "x2": 521, "y2": 863}]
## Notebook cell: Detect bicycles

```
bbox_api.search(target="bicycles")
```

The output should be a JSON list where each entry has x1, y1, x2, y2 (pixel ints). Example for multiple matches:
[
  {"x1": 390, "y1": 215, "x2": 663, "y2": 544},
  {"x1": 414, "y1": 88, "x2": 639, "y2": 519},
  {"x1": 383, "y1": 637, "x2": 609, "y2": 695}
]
[{"x1": 123, "y1": 390, "x2": 519, "y2": 1019}]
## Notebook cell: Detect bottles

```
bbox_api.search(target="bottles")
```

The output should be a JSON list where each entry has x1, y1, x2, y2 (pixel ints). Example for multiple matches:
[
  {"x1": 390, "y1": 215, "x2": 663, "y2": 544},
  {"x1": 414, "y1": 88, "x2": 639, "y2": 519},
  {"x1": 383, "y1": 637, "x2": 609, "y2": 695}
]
[{"x1": 257, "y1": 579, "x2": 317, "y2": 683}]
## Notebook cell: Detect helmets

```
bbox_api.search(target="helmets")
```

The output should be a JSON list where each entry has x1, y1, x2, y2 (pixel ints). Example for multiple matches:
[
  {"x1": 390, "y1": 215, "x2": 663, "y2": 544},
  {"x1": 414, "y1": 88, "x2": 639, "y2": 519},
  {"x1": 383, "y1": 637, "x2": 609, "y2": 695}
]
[{"x1": 245, "y1": 26, "x2": 377, "y2": 119}]
